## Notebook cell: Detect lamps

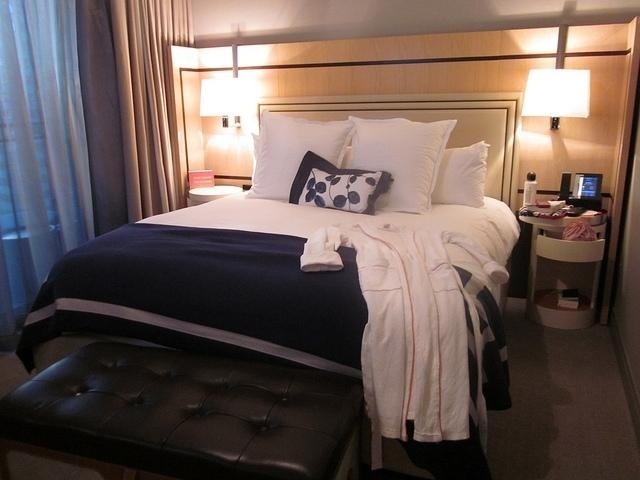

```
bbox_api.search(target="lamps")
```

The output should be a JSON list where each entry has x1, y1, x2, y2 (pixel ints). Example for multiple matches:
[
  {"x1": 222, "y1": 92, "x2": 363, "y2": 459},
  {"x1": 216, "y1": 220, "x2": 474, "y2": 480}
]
[
  {"x1": 200, "y1": 79, "x2": 256, "y2": 127},
  {"x1": 520, "y1": 69, "x2": 591, "y2": 132}
]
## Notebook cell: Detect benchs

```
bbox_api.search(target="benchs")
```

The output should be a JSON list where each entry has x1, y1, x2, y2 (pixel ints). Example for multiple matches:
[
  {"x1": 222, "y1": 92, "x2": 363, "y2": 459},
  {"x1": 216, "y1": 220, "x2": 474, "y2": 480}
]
[{"x1": 1, "y1": 340, "x2": 362, "y2": 480}]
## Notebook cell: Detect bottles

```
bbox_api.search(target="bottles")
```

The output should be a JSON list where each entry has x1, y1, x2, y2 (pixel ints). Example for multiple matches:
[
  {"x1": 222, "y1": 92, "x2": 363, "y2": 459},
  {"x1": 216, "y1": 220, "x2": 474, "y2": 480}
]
[{"x1": 524, "y1": 171, "x2": 537, "y2": 207}]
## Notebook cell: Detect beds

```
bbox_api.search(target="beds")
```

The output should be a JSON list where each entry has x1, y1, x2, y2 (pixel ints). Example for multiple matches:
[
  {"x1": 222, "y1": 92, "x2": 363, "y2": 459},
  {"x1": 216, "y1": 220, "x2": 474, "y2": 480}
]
[{"x1": 17, "y1": 114, "x2": 522, "y2": 478}]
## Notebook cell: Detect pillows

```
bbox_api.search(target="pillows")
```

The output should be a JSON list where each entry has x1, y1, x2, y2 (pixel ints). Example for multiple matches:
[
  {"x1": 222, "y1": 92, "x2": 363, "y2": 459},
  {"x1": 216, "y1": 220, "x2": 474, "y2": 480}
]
[
  {"x1": 347, "y1": 117, "x2": 455, "y2": 216},
  {"x1": 288, "y1": 152, "x2": 394, "y2": 217},
  {"x1": 244, "y1": 110, "x2": 357, "y2": 206},
  {"x1": 246, "y1": 131, "x2": 350, "y2": 200},
  {"x1": 430, "y1": 141, "x2": 490, "y2": 209}
]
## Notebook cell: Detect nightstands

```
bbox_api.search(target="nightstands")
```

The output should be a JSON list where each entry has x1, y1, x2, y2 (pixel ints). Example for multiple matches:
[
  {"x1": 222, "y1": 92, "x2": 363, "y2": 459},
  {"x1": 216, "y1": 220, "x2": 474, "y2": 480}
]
[
  {"x1": 515, "y1": 204, "x2": 608, "y2": 330},
  {"x1": 188, "y1": 185, "x2": 243, "y2": 210}
]
